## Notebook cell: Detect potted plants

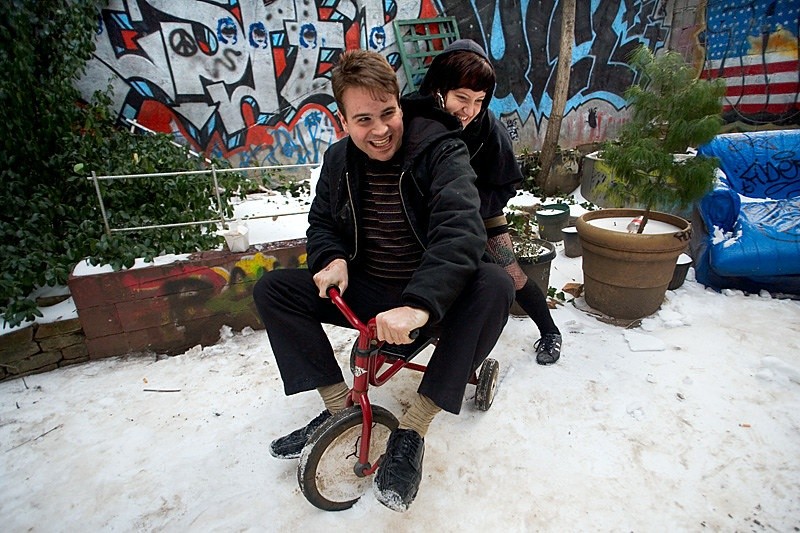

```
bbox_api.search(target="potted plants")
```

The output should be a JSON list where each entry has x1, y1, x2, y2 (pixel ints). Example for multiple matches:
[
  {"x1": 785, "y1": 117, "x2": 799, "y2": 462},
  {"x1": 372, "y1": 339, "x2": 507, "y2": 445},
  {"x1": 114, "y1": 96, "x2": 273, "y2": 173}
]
[
  {"x1": 575, "y1": 44, "x2": 727, "y2": 318},
  {"x1": 505, "y1": 215, "x2": 556, "y2": 314}
]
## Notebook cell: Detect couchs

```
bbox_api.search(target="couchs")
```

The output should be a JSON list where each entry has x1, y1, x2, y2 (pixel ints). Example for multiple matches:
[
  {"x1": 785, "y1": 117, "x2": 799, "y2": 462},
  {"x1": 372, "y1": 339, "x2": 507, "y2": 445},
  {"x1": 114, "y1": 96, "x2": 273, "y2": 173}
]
[{"x1": 690, "y1": 129, "x2": 800, "y2": 296}]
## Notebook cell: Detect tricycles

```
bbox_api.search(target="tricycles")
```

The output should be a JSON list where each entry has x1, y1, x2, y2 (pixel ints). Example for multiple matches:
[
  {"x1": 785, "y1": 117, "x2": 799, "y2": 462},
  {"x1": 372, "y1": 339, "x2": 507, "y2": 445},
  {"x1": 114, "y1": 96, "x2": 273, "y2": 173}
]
[{"x1": 297, "y1": 285, "x2": 499, "y2": 512}]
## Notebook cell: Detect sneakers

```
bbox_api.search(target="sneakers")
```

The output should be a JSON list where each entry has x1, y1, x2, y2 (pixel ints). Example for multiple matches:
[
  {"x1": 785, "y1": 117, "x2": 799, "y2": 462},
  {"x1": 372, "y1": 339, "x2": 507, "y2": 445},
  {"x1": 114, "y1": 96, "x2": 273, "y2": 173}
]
[
  {"x1": 533, "y1": 332, "x2": 562, "y2": 366},
  {"x1": 268, "y1": 408, "x2": 335, "y2": 459},
  {"x1": 372, "y1": 426, "x2": 425, "y2": 514}
]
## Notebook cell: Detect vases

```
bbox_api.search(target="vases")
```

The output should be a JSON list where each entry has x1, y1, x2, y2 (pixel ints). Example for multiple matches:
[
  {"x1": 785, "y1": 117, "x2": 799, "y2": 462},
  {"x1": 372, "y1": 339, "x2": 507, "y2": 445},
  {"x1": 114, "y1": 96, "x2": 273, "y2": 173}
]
[
  {"x1": 562, "y1": 225, "x2": 582, "y2": 257},
  {"x1": 668, "y1": 252, "x2": 693, "y2": 291},
  {"x1": 537, "y1": 203, "x2": 570, "y2": 241}
]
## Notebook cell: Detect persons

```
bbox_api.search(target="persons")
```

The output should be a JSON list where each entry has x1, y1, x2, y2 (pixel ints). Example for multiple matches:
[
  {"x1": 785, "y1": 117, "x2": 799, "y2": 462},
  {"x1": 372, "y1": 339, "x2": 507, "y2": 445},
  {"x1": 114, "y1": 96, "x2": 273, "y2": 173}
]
[
  {"x1": 418, "y1": 39, "x2": 563, "y2": 365},
  {"x1": 253, "y1": 48, "x2": 514, "y2": 512}
]
